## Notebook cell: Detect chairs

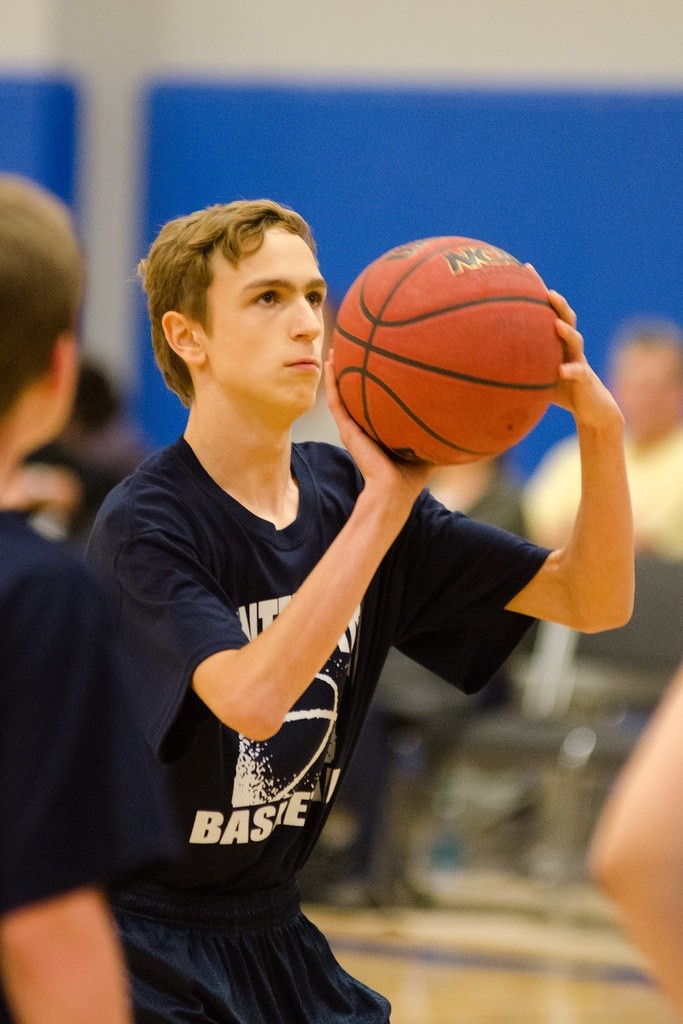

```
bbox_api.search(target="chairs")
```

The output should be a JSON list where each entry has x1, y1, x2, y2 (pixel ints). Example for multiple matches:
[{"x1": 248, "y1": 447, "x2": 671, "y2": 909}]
[{"x1": 306, "y1": 556, "x2": 683, "y2": 911}]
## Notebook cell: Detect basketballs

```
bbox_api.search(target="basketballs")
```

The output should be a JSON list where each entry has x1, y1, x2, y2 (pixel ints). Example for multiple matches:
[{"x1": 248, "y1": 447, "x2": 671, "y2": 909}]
[{"x1": 333, "y1": 232, "x2": 567, "y2": 467}]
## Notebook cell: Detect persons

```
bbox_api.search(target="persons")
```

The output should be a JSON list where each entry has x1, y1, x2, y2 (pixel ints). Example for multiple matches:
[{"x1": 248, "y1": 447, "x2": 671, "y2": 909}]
[
  {"x1": 586, "y1": 658, "x2": 682, "y2": 1022},
  {"x1": 1, "y1": 348, "x2": 157, "y2": 555},
  {"x1": 296, "y1": 456, "x2": 538, "y2": 909},
  {"x1": 518, "y1": 312, "x2": 682, "y2": 885},
  {"x1": 1, "y1": 171, "x2": 136, "y2": 1024},
  {"x1": 80, "y1": 198, "x2": 634, "y2": 1024}
]
